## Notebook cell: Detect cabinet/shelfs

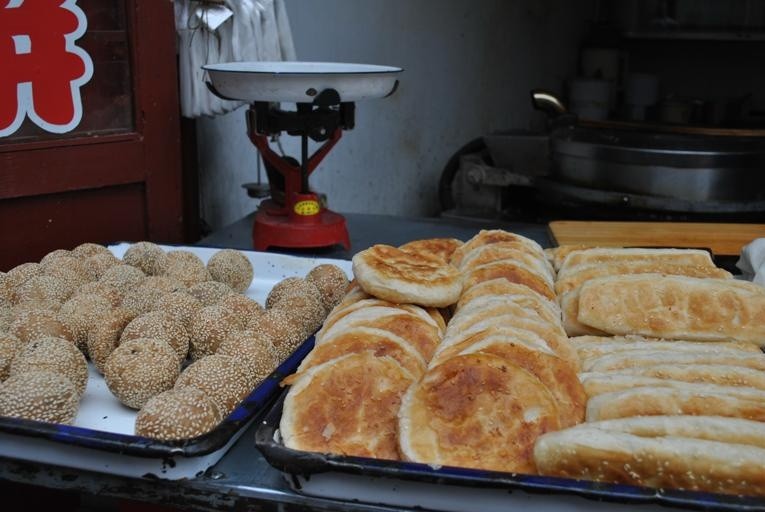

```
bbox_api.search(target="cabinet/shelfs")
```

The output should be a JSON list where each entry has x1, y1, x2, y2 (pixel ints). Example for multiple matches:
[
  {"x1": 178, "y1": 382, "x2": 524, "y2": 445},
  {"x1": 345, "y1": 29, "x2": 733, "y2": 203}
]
[{"x1": 569, "y1": 28, "x2": 765, "y2": 137}]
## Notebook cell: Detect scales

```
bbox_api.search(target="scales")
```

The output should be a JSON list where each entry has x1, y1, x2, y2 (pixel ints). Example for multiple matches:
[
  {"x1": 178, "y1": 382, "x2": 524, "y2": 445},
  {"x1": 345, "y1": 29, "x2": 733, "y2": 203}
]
[{"x1": 200, "y1": 61, "x2": 403, "y2": 253}]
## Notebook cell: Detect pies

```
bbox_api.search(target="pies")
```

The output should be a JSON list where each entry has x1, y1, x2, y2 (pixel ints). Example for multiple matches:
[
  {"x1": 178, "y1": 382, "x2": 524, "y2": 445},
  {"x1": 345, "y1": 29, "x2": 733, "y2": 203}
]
[{"x1": 278, "y1": 228, "x2": 765, "y2": 496}]
